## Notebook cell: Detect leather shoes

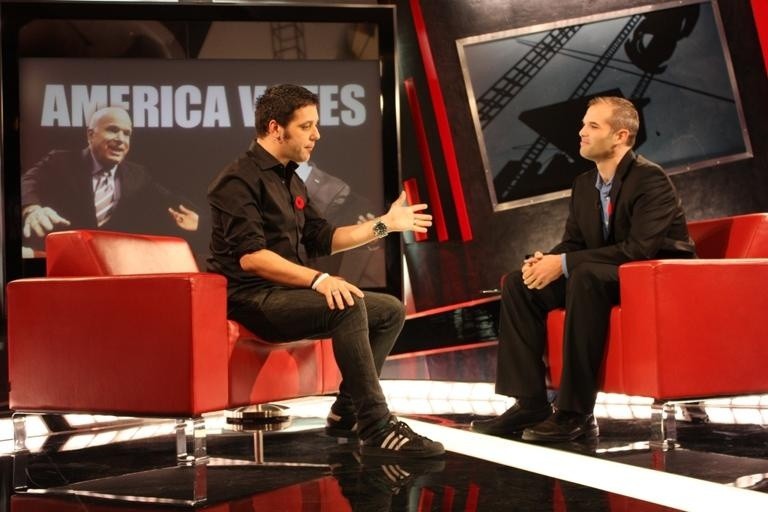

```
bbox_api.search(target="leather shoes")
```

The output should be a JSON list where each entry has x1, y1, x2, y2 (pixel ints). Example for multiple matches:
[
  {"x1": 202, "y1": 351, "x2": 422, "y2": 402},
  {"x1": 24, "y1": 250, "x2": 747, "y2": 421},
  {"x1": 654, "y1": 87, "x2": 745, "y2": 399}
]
[
  {"x1": 470, "y1": 400, "x2": 553, "y2": 436},
  {"x1": 521, "y1": 409, "x2": 599, "y2": 442}
]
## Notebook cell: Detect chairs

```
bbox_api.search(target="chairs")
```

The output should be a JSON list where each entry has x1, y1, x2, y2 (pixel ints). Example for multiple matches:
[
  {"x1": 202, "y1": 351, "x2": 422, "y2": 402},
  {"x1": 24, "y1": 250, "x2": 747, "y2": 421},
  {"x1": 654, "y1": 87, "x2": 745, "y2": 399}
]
[
  {"x1": 8, "y1": 229, "x2": 346, "y2": 508},
  {"x1": 499, "y1": 213, "x2": 767, "y2": 489}
]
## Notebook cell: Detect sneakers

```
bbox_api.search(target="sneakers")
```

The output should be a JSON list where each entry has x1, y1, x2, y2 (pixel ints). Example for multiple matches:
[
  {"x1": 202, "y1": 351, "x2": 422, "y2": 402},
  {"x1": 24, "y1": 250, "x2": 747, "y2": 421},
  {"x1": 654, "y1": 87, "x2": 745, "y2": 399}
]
[
  {"x1": 360, "y1": 416, "x2": 445, "y2": 459},
  {"x1": 325, "y1": 408, "x2": 360, "y2": 439},
  {"x1": 361, "y1": 457, "x2": 446, "y2": 495}
]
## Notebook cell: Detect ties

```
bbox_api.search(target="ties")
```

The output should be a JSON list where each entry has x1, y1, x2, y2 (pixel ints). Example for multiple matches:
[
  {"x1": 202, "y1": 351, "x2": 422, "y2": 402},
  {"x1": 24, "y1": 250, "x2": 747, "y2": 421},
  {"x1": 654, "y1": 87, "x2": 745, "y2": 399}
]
[{"x1": 94, "y1": 171, "x2": 114, "y2": 226}]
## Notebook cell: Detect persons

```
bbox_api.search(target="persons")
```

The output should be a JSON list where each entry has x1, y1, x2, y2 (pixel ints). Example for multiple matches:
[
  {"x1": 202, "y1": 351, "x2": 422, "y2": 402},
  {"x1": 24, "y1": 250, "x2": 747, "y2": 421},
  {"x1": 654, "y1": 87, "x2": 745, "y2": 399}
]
[
  {"x1": 205, "y1": 83, "x2": 448, "y2": 460},
  {"x1": 21, "y1": 106, "x2": 173, "y2": 251},
  {"x1": 323, "y1": 446, "x2": 446, "y2": 511},
  {"x1": 468, "y1": 96, "x2": 698, "y2": 444},
  {"x1": 168, "y1": 153, "x2": 387, "y2": 275}
]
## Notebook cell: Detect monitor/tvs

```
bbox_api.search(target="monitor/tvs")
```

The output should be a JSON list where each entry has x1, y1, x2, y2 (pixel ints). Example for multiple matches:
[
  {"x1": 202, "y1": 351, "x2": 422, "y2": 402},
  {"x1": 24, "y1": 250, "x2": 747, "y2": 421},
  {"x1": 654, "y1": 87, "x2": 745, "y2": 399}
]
[{"x1": 455, "y1": 0, "x2": 754, "y2": 212}]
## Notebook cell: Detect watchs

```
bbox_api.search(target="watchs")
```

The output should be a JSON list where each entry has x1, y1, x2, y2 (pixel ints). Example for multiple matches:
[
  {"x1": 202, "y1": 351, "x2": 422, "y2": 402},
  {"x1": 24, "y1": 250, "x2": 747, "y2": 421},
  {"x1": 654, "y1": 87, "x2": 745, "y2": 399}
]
[{"x1": 370, "y1": 215, "x2": 388, "y2": 240}]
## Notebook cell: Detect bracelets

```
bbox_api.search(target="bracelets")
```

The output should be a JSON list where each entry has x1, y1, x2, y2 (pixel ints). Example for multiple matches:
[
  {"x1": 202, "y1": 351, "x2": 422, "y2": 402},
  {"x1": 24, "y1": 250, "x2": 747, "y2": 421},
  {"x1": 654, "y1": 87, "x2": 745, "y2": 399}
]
[{"x1": 311, "y1": 270, "x2": 329, "y2": 291}]
